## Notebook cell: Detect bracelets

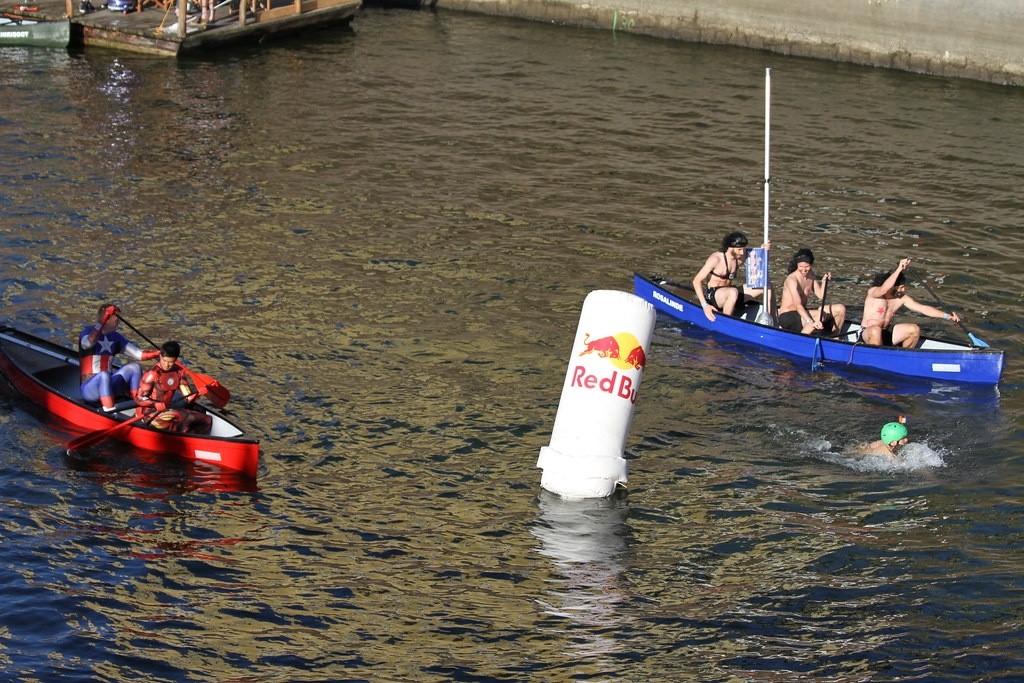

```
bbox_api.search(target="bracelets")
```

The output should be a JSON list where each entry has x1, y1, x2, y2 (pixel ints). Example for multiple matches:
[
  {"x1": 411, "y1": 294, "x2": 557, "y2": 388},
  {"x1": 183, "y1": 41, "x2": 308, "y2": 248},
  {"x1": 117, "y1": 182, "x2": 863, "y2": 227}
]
[
  {"x1": 807, "y1": 318, "x2": 812, "y2": 323},
  {"x1": 944, "y1": 314, "x2": 950, "y2": 320},
  {"x1": 701, "y1": 302, "x2": 707, "y2": 307},
  {"x1": 95, "y1": 323, "x2": 103, "y2": 332}
]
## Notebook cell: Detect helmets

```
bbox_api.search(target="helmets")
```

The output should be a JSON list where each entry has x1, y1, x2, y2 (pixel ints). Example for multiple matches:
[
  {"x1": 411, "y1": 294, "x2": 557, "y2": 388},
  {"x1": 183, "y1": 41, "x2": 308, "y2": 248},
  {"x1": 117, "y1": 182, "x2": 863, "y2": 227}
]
[{"x1": 880, "y1": 421, "x2": 908, "y2": 445}]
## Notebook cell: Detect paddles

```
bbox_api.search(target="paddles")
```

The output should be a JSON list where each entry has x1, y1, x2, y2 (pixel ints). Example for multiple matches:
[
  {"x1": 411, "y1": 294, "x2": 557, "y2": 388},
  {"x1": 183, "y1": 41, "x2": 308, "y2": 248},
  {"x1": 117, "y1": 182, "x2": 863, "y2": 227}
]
[
  {"x1": 66, "y1": 380, "x2": 220, "y2": 451},
  {"x1": 811, "y1": 272, "x2": 828, "y2": 370},
  {"x1": 112, "y1": 312, "x2": 230, "y2": 408},
  {"x1": 907, "y1": 264, "x2": 990, "y2": 347}
]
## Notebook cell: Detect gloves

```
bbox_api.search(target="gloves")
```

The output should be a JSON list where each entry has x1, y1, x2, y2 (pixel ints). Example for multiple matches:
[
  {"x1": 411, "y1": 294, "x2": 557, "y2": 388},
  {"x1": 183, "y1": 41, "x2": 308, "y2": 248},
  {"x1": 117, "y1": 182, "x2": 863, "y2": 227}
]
[
  {"x1": 104, "y1": 305, "x2": 116, "y2": 319},
  {"x1": 198, "y1": 384, "x2": 212, "y2": 396},
  {"x1": 154, "y1": 402, "x2": 166, "y2": 412}
]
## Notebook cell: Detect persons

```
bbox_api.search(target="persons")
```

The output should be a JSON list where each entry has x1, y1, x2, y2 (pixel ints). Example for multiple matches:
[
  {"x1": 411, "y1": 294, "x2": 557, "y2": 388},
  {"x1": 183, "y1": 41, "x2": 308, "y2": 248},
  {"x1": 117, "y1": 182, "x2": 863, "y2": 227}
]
[
  {"x1": 78, "y1": 303, "x2": 161, "y2": 416},
  {"x1": 136, "y1": 341, "x2": 212, "y2": 435},
  {"x1": 779, "y1": 248, "x2": 846, "y2": 340},
  {"x1": 859, "y1": 258, "x2": 960, "y2": 348},
  {"x1": 693, "y1": 231, "x2": 772, "y2": 323},
  {"x1": 173, "y1": 0, "x2": 264, "y2": 24},
  {"x1": 874, "y1": 422, "x2": 909, "y2": 452}
]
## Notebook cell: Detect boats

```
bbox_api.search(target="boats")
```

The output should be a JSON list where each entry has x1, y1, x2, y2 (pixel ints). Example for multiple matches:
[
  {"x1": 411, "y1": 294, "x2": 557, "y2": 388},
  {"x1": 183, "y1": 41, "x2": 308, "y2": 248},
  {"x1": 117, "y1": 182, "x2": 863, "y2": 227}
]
[
  {"x1": 632, "y1": 271, "x2": 1005, "y2": 385},
  {"x1": 0, "y1": 16, "x2": 70, "y2": 48},
  {"x1": 0, "y1": 324, "x2": 261, "y2": 479}
]
